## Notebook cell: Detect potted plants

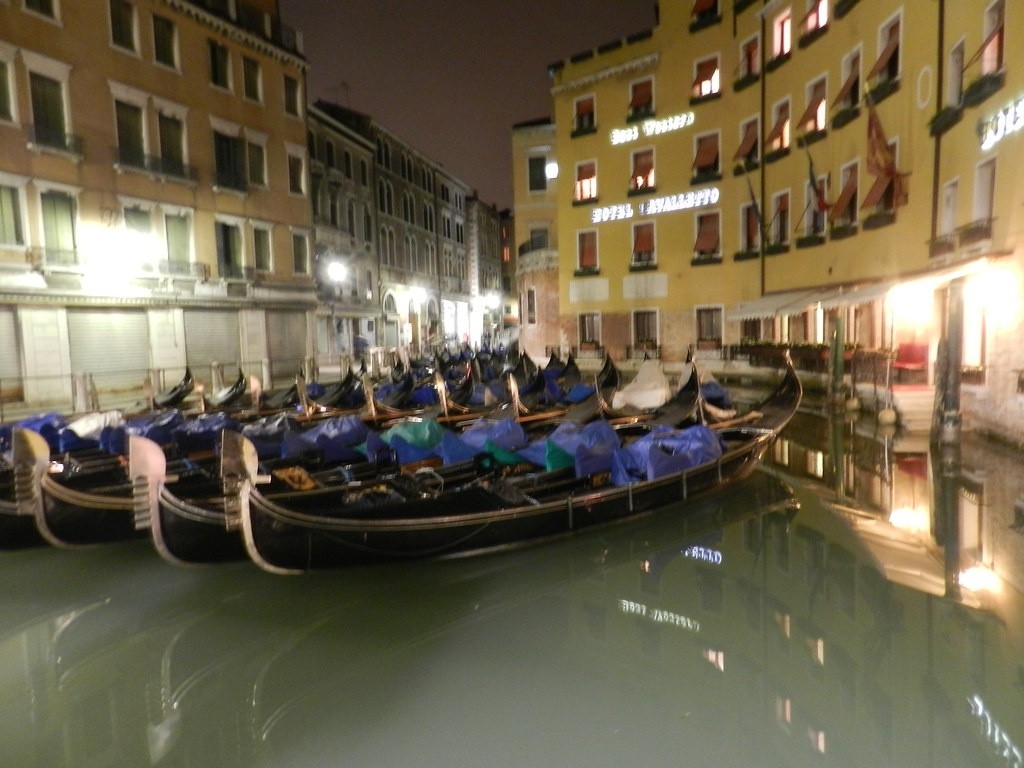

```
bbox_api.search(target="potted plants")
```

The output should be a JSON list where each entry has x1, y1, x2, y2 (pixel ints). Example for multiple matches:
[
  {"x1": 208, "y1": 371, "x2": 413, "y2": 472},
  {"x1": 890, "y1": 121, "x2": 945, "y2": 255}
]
[{"x1": 740, "y1": 341, "x2": 853, "y2": 360}]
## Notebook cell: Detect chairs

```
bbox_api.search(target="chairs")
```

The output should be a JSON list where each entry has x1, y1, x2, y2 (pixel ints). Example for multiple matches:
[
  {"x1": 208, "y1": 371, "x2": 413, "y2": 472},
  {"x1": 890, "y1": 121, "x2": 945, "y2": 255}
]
[{"x1": 892, "y1": 344, "x2": 926, "y2": 383}]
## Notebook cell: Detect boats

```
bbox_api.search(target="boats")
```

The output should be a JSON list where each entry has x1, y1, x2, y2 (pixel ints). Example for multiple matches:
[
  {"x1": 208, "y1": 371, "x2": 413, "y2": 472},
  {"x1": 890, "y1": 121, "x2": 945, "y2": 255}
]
[
  {"x1": 0, "y1": 342, "x2": 736, "y2": 562},
  {"x1": 219, "y1": 348, "x2": 803, "y2": 577}
]
